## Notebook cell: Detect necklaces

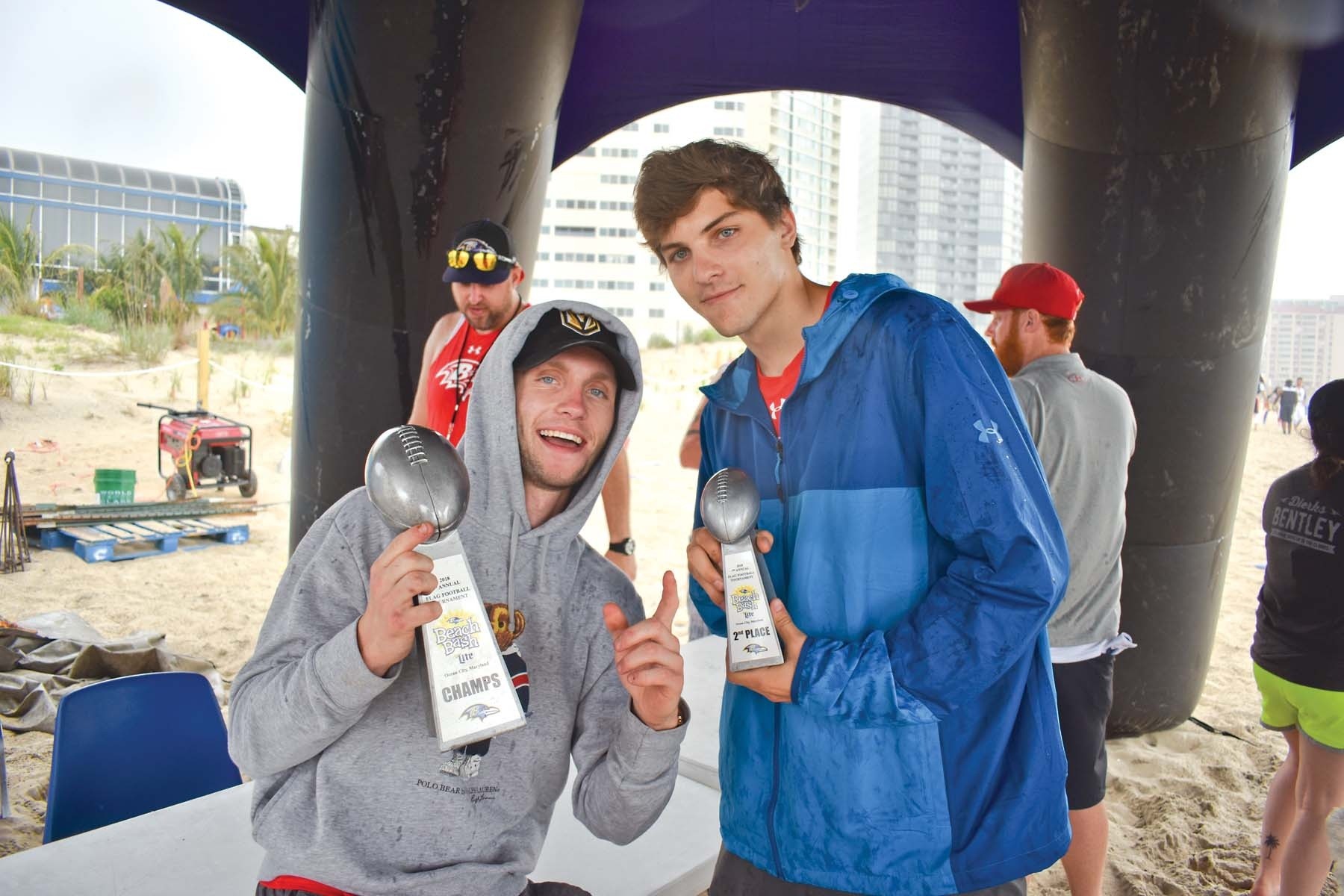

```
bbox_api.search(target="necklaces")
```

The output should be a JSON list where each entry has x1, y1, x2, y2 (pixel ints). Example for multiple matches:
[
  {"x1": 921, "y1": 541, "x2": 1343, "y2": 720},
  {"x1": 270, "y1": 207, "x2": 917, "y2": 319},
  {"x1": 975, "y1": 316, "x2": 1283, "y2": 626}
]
[{"x1": 446, "y1": 296, "x2": 521, "y2": 440}]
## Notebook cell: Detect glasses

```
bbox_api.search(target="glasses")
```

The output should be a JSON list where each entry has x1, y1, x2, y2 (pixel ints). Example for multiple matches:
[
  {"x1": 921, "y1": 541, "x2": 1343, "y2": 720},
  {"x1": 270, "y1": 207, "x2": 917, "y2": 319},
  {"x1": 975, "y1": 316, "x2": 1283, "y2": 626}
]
[{"x1": 447, "y1": 247, "x2": 520, "y2": 271}]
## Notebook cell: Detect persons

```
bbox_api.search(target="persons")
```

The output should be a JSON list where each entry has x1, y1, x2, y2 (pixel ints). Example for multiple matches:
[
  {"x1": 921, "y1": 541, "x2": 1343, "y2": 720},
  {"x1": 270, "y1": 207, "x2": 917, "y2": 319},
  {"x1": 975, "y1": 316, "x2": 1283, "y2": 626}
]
[
  {"x1": 964, "y1": 263, "x2": 1138, "y2": 896},
  {"x1": 631, "y1": 137, "x2": 1071, "y2": 895},
  {"x1": 228, "y1": 302, "x2": 693, "y2": 896},
  {"x1": 1254, "y1": 376, "x2": 1321, "y2": 434},
  {"x1": 1249, "y1": 379, "x2": 1344, "y2": 896},
  {"x1": 406, "y1": 220, "x2": 638, "y2": 586}
]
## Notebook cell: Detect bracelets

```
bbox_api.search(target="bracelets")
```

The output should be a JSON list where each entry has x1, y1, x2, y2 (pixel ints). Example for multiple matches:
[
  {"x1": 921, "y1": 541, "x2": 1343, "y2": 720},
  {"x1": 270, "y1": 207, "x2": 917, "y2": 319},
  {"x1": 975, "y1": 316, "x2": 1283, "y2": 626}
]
[{"x1": 677, "y1": 708, "x2": 684, "y2": 727}]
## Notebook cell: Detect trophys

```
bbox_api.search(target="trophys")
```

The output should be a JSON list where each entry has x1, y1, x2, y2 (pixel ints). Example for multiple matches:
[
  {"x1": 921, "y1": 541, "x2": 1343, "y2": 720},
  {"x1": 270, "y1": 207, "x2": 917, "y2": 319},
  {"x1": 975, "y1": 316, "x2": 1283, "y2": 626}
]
[
  {"x1": 700, "y1": 467, "x2": 786, "y2": 672},
  {"x1": 365, "y1": 423, "x2": 527, "y2": 755}
]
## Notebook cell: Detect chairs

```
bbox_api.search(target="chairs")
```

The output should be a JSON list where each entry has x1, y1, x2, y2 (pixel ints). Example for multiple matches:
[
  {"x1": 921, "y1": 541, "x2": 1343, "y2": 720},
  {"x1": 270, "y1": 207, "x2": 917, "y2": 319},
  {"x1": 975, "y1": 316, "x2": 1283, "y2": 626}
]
[{"x1": 42, "y1": 672, "x2": 244, "y2": 842}]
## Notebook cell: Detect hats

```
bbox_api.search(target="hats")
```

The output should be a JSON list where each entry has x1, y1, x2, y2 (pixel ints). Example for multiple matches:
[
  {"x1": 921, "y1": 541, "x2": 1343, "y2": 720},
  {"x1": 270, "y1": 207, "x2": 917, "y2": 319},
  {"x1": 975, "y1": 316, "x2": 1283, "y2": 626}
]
[
  {"x1": 442, "y1": 218, "x2": 516, "y2": 284},
  {"x1": 513, "y1": 310, "x2": 637, "y2": 394},
  {"x1": 962, "y1": 261, "x2": 1083, "y2": 322}
]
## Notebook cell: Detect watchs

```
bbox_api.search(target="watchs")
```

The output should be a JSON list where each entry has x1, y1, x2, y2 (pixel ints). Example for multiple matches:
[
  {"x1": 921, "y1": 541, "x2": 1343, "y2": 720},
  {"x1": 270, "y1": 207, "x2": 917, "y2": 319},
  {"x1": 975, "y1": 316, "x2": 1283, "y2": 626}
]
[{"x1": 608, "y1": 537, "x2": 634, "y2": 556}]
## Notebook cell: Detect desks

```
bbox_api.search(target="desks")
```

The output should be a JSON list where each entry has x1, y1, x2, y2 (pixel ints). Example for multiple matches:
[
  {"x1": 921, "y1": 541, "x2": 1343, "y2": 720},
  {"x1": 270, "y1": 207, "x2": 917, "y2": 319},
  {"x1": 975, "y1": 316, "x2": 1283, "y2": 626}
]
[
  {"x1": 0, "y1": 749, "x2": 721, "y2": 896},
  {"x1": 676, "y1": 634, "x2": 728, "y2": 791}
]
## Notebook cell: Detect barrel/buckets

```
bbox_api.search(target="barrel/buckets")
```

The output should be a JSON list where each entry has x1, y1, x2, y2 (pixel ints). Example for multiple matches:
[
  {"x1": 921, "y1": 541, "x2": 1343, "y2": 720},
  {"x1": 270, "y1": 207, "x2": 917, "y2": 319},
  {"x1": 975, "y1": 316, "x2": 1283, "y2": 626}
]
[{"x1": 94, "y1": 469, "x2": 136, "y2": 505}]
